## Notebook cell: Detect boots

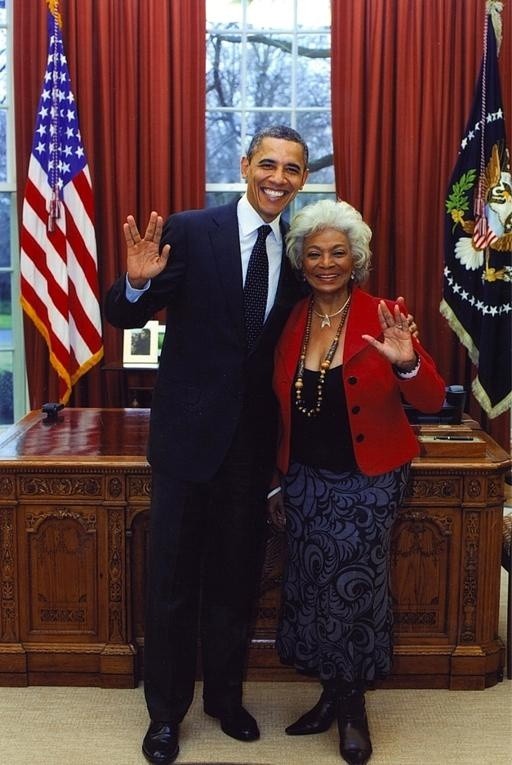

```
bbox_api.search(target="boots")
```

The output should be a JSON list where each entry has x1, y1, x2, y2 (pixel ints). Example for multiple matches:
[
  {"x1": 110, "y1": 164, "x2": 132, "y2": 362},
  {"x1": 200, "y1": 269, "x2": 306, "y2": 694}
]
[
  {"x1": 335, "y1": 691, "x2": 372, "y2": 765},
  {"x1": 286, "y1": 690, "x2": 339, "y2": 736}
]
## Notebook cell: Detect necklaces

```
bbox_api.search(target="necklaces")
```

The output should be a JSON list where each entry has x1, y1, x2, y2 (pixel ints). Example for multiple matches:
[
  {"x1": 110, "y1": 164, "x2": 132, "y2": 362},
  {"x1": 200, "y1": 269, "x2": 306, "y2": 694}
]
[
  {"x1": 294, "y1": 300, "x2": 349, "y2": 418},
  {"x1": 312, "y1": 292, "x2": 351, "y2": 328}
]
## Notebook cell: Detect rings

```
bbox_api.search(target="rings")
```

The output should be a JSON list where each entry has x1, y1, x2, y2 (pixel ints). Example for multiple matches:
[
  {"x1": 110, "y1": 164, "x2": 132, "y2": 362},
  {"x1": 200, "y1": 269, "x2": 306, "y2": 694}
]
[{"x1": 396, "y1": 325, "x2": 402, "y2": 329}]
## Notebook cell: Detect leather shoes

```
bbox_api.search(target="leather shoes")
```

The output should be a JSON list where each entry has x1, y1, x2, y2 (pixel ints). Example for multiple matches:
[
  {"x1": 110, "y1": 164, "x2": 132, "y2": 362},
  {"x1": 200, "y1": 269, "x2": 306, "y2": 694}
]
[
  {"x1": 142, "y1": 720, "x2": 180, "y2": 764},
  {"x1": 203, "y1": 701, "x2": 259, "y2": 741}
]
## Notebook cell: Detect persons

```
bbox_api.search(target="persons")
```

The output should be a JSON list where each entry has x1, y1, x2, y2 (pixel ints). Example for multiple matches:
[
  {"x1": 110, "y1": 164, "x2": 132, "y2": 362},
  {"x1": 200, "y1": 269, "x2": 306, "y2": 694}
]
[
  {"x1": 105, "y1": 125, "x2": 421, "y2": 764},
  {"x1": 267, "y1": 199, "x2": 446, "y2": 765}
]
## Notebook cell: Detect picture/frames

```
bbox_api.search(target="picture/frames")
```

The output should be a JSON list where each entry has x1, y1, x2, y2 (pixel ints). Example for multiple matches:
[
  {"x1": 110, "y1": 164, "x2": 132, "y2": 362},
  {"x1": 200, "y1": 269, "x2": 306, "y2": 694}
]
[{"x1": 122, "y1": 320, "x2": 159, "y2": 364}]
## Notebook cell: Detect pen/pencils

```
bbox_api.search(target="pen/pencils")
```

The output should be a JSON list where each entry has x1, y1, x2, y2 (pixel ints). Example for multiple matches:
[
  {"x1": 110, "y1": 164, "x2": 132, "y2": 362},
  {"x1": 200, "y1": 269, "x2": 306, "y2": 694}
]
[{"x1": 436, "y1": 436, "x2": 473, "y2": 440}]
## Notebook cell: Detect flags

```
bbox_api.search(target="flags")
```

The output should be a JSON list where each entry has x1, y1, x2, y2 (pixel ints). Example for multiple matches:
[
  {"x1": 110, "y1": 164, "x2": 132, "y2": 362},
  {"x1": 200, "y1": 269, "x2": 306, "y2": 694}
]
[
  {"x1": 438, "y1": 0, "x2": 512, "y2": 420},
  {"x1": 19, "y1": 0, "x2": 105, "y2": 406}
]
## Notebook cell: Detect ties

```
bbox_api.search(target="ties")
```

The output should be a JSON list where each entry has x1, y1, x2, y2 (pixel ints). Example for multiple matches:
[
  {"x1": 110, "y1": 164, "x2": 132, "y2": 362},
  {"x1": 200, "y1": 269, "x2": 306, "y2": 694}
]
[{"x1": 245, "y1": 226, "x2": 273, "y2": 356}]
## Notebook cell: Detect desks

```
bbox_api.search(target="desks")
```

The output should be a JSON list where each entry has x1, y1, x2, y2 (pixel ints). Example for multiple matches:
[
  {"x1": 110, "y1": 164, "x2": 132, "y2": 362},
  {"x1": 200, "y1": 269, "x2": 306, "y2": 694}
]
[{"x1": 1, "y1": 406, "x2": 509, "y2": 691}]
